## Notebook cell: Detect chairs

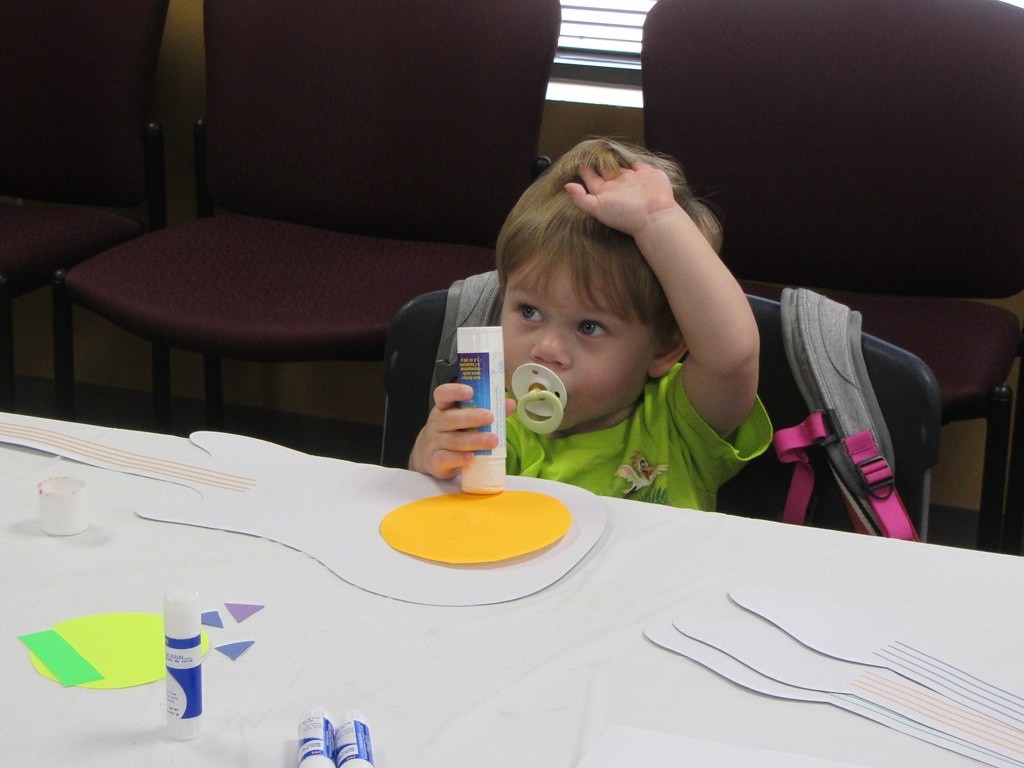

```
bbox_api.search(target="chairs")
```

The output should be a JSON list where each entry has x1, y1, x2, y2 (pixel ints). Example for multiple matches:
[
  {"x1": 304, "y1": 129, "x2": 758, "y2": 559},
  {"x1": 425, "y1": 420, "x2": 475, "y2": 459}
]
[
  {"x1": 642, "y1": 0, "x2": 1021, "y2": 550},
  {"x1": 0, "y1": 0, "x2": 180, "y2": 444},
  {"x1": 50, "y1": 0, "x2": 563, "y2": 468}
]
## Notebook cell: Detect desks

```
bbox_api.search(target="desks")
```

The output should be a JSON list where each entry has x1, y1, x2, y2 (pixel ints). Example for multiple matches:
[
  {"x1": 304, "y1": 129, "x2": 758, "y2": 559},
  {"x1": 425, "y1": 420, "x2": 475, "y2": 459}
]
[{"x1": 0, "y1": 407, "x2": 1023, "y2": 764}]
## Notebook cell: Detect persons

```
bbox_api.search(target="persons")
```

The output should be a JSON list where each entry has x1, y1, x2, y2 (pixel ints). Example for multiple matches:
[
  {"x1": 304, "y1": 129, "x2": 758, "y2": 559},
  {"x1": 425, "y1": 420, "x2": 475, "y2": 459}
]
[{"x1": 409, "y1": 138, "x2": 774, "y2": 516}]
